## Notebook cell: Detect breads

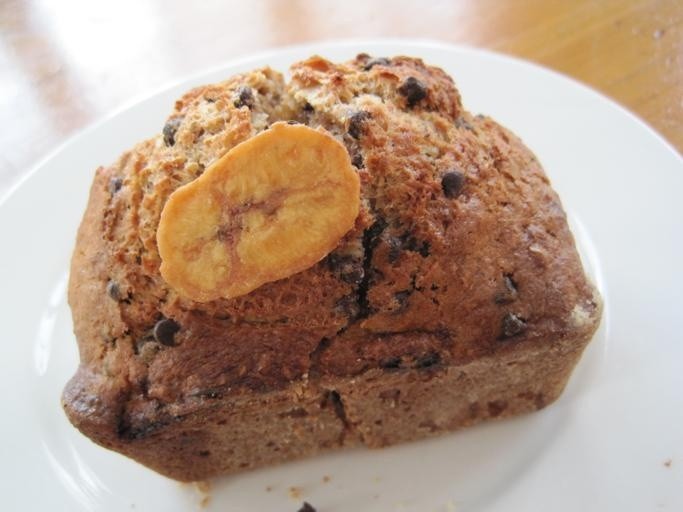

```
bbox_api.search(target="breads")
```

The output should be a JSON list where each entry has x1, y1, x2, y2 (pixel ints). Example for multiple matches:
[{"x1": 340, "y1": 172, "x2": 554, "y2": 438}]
[{"x1": 61, "y1": 53, "x2": 602, "y2": 480}]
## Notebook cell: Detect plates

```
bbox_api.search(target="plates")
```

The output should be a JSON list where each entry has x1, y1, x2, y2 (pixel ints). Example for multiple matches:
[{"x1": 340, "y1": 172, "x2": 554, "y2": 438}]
[{"x1": 1, "y1": 36, "x2": 680, "y2": 512}]
